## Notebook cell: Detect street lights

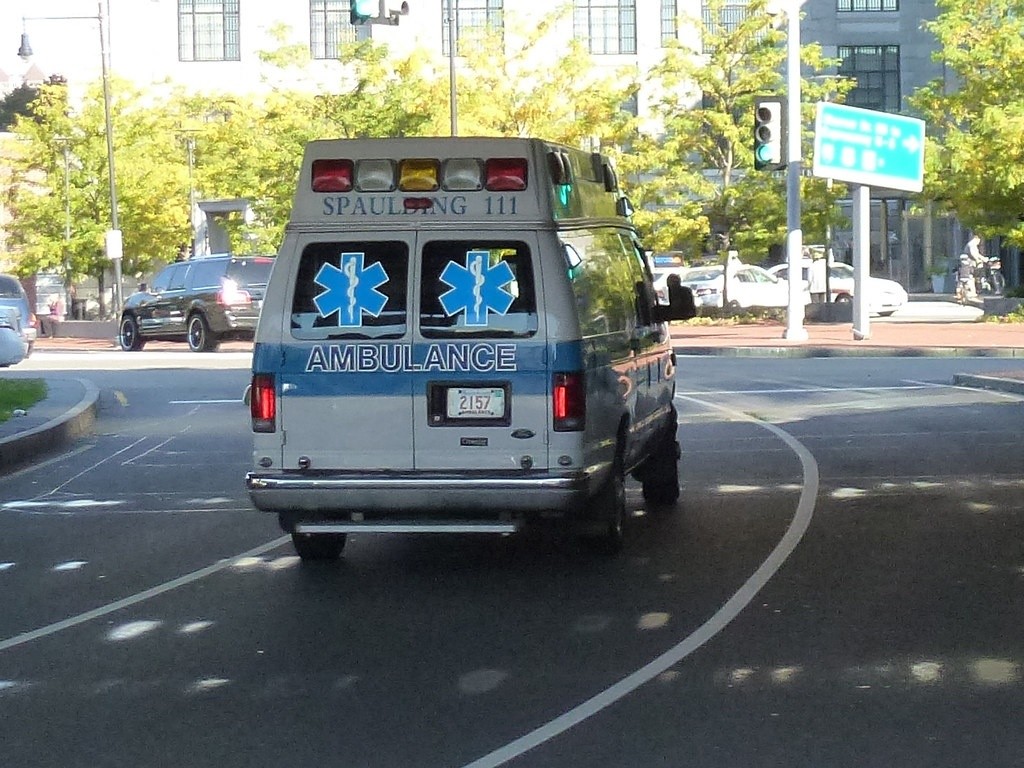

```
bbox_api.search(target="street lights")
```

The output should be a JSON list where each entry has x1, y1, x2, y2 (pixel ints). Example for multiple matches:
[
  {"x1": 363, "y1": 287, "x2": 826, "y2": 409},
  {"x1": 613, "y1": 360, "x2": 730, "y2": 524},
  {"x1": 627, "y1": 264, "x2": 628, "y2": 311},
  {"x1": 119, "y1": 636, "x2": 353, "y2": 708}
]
[
  {"x1": 53, "y1": 137, "x2": 79, "y2": 270},
  {"x1": 17, "y1": 3, "x2": 124, "y2": 322},
  {"x1": 176, "y1": 128, "x2": 207, "y2": 255}
]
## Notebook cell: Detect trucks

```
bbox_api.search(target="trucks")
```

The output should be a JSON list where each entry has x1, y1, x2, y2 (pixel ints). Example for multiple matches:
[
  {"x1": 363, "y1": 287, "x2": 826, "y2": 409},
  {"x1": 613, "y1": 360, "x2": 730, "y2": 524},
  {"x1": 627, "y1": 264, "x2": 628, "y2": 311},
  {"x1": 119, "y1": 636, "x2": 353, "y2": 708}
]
[{"x1": 20, "y1": 274, "x2": 68, "y2": 315}]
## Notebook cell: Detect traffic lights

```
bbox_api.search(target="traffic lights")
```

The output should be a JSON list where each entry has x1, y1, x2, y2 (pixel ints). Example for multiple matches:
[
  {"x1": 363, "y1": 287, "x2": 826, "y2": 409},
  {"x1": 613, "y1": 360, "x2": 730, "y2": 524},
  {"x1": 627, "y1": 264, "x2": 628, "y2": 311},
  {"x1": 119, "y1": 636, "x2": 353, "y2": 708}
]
[
  {"x1": 754, "y1": 96, "x2": 788, "y2": 170},
  {"x1": 350, "y1": 0, "x2": 410, "y2": 26}
]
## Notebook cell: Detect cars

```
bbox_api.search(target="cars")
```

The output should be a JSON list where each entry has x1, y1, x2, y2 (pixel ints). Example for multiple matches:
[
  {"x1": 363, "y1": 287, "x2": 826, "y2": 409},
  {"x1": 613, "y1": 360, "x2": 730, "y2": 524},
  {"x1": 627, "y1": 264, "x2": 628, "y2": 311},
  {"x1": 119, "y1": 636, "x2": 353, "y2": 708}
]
[
  {"x1": 662, "y1": 264, "x2": 813, "y2": 309},
  {"x1": 0, "y1": 273, "x2": 38, "y2": 367},
  {"x1": 768, "y1": 260, "x2": 908, "y2": 316},
  {"x1": 652, "y1": 267, "x2": 688, "y2": 305}
]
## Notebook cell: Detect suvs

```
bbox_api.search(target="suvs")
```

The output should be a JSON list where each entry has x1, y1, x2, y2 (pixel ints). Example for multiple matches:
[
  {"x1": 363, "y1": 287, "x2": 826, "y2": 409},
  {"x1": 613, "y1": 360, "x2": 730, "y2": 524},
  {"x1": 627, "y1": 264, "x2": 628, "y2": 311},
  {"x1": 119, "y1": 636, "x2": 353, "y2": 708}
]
[{"x1": 119, "y1": 256, "x2": 276, "y2": 352}]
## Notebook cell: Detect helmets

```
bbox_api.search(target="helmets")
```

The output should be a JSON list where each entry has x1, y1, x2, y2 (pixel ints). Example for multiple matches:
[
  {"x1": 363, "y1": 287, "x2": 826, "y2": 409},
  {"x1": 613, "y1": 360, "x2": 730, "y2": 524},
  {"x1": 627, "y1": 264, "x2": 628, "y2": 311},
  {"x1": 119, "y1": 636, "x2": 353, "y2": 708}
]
[{"x1": 960, "y1": 254, "x2": 969, "y2": 260}]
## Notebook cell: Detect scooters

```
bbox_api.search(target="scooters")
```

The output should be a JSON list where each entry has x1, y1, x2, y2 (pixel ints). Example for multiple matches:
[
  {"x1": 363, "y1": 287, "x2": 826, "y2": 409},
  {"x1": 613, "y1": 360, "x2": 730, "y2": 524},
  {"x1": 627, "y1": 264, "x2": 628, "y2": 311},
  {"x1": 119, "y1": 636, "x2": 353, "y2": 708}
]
[{"x1": 952, "y1": 257, "x2": 1005, "y2": 296}]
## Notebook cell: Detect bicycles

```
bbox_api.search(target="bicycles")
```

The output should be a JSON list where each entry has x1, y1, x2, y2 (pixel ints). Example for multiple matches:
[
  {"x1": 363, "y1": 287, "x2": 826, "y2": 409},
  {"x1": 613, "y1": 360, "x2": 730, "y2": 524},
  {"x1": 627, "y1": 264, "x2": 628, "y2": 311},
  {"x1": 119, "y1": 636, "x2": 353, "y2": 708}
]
[{"x1": 954, "y1": 271, "x2": 973, "y2": 306}]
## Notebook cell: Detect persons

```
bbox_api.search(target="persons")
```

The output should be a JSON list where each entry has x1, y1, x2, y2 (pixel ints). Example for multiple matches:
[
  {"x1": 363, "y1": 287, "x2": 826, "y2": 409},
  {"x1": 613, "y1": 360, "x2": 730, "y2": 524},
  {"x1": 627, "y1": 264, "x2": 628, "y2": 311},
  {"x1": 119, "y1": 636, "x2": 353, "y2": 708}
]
[
  {"x1": 667, "y1": 274, "x2": 696, "y2": 321},
  {"x1": 808, "y1": 253, "x2": 827, "y2": 303},
  {"x1": 43, "y1": 295, "x2": 64, "y2": 338},
  {"x1": 953, "y1": 235, "x2": 984, "y2": 299}
]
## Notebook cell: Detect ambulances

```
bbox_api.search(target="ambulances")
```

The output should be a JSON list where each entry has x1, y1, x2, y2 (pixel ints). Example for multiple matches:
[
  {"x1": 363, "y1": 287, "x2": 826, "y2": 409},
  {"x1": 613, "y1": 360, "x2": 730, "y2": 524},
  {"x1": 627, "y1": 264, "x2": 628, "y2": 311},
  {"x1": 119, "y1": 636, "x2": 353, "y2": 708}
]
[{"x1": 242, "y1": 136, "x2": 697, "y2": 560}]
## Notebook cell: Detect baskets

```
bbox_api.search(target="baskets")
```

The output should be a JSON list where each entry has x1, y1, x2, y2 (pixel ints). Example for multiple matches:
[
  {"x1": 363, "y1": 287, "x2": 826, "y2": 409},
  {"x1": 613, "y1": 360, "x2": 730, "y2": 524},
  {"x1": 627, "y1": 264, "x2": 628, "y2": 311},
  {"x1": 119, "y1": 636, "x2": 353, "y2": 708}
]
[{"x1": 989, "y1": 259, "x2": 1001, "y2": 270}]
